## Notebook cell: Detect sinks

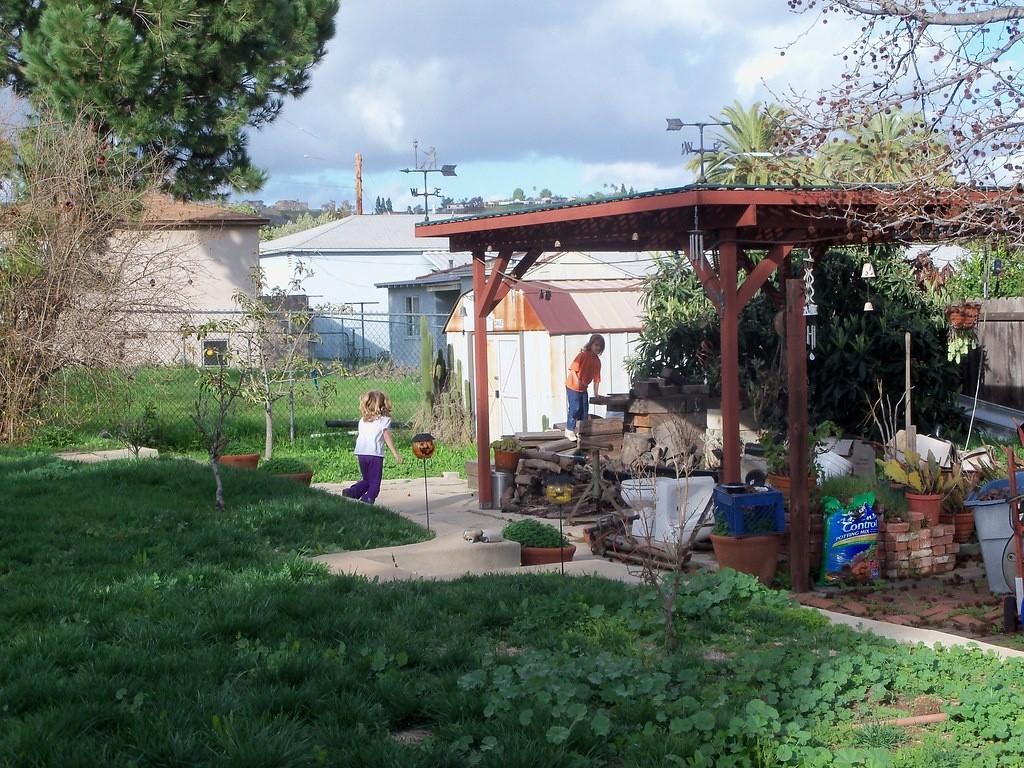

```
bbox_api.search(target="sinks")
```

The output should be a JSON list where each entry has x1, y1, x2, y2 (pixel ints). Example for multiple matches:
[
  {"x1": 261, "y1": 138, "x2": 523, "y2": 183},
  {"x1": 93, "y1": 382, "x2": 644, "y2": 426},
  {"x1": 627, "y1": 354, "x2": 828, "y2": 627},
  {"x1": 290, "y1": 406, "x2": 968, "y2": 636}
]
[{"x1": 655, "y1": 476, "x2": 714, "y2": 542}]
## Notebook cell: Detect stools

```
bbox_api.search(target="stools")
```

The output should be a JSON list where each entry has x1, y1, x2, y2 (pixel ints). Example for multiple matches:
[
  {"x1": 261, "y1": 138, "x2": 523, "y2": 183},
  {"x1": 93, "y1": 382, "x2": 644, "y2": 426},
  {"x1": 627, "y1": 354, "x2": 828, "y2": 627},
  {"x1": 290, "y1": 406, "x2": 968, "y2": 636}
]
[{"x1": 712, "y1": 485, "x2": 786, "y2": 536}]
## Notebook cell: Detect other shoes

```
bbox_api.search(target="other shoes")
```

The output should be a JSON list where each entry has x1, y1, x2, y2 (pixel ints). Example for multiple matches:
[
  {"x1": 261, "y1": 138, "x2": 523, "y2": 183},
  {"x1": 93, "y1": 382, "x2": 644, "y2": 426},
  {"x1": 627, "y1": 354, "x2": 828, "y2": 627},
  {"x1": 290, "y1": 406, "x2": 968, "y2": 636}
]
[{"x1": 342, "y1": 489, "x2": 350, "y2": 498}]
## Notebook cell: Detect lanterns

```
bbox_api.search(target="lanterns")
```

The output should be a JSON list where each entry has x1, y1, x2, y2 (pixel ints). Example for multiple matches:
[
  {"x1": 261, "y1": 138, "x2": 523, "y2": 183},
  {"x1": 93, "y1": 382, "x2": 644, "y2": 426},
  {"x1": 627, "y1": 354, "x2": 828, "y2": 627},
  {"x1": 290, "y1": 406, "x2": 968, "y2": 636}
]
[
  {"x1": 543, "y1": 475, "x2": 573, "y2": 504},
  {"x1": 411, "y1": 434, "x2": 436, "y2": 458}
]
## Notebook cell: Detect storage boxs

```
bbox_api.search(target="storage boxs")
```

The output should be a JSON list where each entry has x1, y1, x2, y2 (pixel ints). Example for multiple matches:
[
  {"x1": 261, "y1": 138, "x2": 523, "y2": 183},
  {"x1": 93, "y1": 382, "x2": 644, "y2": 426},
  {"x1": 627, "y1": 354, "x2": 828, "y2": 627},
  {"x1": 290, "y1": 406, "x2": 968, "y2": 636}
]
[
  {"x1": 821, "y1": 439, "x2": 876, "y2": 478},
  {"x1": 886, "y1": 430, "x2": 952, "y2": 468}
]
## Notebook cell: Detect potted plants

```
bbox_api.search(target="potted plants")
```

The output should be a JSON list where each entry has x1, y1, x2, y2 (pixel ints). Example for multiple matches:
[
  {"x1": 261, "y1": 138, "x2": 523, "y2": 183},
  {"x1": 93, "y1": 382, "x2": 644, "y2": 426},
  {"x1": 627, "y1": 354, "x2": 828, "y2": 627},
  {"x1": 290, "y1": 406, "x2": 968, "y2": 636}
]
[
  {"x1": 754, "y1": 420, "x2": 843, "y2": 503},
  {"x1": 874, "y1": 449, "x2": 964, "y2": 526},
  {"x1": 939, "y1": 463, "x2": 980, "y2": 544},
  {"x1": 500, "y1": 518, "x2": 577, "y2": 566},
  {"x1": 257, "y1": 457, "x2": 314, "y2": 487},
  {"x1": 709, "y1": 509, "x2": 785, "y2": 587},
  {"x1": 489, "y1": 438, "x2": 525, "y2": 474},
  {"x1": 218, "y1": 442, "x2": 261, "y2": 468}
]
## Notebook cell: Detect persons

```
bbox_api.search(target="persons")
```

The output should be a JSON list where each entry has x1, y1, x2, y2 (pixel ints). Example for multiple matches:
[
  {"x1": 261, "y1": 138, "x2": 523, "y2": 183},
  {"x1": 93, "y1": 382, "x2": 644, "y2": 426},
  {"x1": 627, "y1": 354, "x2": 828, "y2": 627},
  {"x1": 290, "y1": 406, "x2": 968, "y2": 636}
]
[
  {"x1": 564, "y1": 335, "x2": 605, "y2": 442},
  {"x1": 341, "y1": 390, "x2": 404, "y2": 504}
]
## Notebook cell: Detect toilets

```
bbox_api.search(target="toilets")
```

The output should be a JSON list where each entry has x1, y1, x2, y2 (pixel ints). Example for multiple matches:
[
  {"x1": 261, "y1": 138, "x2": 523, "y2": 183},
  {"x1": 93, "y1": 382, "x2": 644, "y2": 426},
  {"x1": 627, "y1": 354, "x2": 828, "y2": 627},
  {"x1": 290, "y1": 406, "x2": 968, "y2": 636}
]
[{"x1": 621, "y1": 477, "x2": 673, "y2": 536}]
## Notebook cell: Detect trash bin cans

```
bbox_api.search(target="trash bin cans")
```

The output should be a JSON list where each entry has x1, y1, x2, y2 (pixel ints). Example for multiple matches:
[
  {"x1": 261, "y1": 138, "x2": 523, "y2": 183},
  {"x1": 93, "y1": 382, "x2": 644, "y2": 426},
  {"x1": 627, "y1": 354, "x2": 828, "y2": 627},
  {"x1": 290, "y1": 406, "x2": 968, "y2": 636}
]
[{"x1": 962, "y1": 475, "x2": 1024, "y2": 594}]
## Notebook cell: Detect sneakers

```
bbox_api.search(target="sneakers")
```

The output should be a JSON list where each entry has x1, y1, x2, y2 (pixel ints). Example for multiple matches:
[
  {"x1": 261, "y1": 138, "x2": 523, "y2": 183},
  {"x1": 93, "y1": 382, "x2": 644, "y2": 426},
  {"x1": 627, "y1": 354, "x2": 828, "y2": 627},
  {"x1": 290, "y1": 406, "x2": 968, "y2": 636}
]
[{"x1": 565, "y1": 428, "x2": 577, "y2": 441}]
